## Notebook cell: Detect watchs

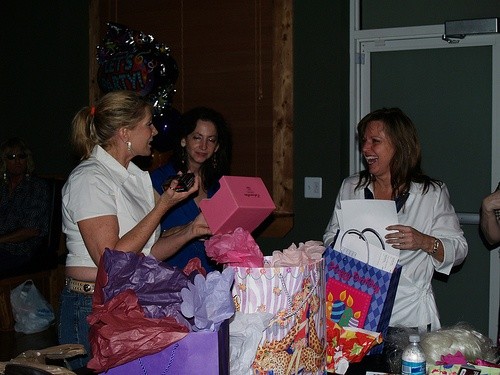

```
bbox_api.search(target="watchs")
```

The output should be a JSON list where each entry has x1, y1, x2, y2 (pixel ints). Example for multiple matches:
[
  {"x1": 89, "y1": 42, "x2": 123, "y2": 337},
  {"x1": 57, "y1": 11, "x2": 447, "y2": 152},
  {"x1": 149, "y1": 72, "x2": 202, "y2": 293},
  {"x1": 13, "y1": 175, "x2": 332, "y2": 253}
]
[{"x1": 427, "y1": 236, "x2": 441, "y2": 256}]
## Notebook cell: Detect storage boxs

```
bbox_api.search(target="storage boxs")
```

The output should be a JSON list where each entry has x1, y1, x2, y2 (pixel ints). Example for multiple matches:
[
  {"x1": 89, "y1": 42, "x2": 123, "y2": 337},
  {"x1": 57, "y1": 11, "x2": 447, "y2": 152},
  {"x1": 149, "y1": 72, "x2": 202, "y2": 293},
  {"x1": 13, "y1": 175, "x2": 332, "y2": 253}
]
[{"x1": 199, "y1": 175, "x2": 275, "y2": 235}]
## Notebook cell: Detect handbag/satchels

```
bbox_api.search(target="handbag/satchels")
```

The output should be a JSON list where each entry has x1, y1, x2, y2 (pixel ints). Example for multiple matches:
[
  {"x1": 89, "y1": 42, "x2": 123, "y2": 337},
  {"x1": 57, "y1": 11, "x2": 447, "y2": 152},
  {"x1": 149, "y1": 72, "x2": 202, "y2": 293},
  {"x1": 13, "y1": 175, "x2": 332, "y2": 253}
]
[
  {"x1": 9, "y1": 280, "x2": 56, "y2": 334},
  {"x1": 0, "y1": 344, "x2": 87, "y2": 375},
  {"x1": 98, "y1": 330, "x2": 220, "y2": 375},
  {"x1": 322, "y1": 228, "x2": 403, "y2": 356},
  {"x1": 223, "y1": 255, "x2": 325, "y2": 375}
]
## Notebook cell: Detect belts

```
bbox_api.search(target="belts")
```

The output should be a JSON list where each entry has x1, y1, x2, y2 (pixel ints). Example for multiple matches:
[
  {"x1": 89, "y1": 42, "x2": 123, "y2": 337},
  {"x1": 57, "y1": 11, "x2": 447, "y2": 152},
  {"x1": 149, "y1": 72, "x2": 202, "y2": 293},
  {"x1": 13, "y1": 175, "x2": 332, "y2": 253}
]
[{"x1": 65, "y1": 278, "x2": 94, "y2": 293}]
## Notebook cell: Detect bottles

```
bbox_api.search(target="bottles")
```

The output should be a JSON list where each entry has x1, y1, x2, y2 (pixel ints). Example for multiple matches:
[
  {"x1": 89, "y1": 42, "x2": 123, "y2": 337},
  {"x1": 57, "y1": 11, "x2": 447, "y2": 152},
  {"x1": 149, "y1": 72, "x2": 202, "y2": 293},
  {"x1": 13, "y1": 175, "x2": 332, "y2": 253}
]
[{"x1": 401, "y1": 334, "x2": 426, "y2": 375}]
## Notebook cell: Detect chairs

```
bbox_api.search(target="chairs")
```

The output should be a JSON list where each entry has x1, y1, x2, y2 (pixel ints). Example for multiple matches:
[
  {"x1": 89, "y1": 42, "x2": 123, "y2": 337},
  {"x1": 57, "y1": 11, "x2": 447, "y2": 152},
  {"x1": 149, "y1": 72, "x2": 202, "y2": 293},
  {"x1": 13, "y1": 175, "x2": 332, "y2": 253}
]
[{"x1": 0, "y1": 164, "x2": 59, "y2": 332}]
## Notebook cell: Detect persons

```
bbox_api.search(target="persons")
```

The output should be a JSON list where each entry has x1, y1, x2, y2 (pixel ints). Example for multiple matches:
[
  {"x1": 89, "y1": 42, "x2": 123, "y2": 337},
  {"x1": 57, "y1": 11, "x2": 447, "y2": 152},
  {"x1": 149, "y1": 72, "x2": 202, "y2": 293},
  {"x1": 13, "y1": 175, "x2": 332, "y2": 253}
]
[
  {"x1": 320, "y1": 109, "x2": 469, "y2": 336},
  {"x1": 150, "y1": 110, "x2": 228, "y2": 274},
  {"x1": 57, "y1": 90, "x2": 200, "y2": 375},
  {"x1": 0, "y1": 140, "x2": 49, "y2": 279},
  {"x1": 478, "y1": 180, "x2": 500, "y2": 249}
]
